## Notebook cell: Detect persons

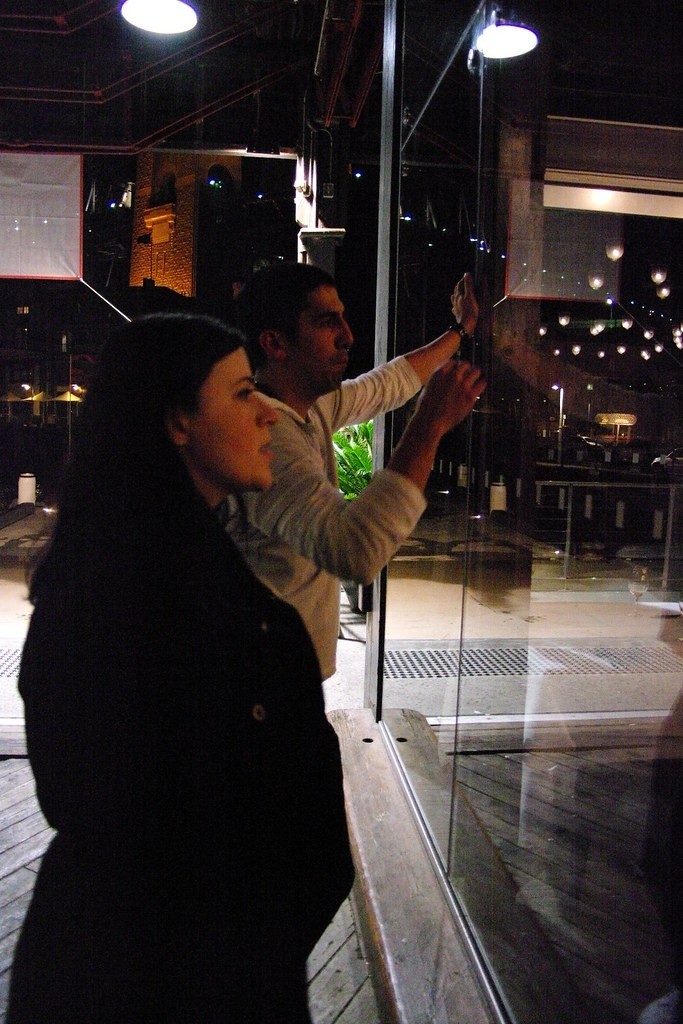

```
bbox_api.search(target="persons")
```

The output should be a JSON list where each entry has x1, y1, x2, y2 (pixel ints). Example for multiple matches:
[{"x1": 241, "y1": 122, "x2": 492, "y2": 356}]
[
  {"x1": 203, "y1": 164, "x2": 243, "y2": 228},
  {"x1": 5, "y1": 310, "x2": 356, "y2": 1023},
  {"x1": 219, "y1": 263, "x2": 488, "y2": 678}
]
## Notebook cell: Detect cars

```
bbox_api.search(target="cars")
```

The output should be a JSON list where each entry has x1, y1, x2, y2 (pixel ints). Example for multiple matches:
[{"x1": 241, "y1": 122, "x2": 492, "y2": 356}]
[
  {"x1": 564, "y1": 434, "x2": 607, "y2": 464},
  {"x1": 650, "y1": 446, "x2": 683, "y2": 476},
  {"x1": 611, "y1": 438, "x2": 654, "y2": 470}
]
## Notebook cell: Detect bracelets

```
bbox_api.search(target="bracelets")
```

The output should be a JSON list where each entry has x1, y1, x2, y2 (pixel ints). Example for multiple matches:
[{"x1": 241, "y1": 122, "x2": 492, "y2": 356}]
[{"x1": 448, "y1": 322, "x2": 469, "y2": 341}]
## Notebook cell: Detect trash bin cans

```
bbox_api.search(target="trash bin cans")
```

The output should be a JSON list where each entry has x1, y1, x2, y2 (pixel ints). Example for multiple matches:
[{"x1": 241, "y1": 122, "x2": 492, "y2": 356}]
[{"x1": 17, "y1": 473, "x2": 37, "y2": 507}]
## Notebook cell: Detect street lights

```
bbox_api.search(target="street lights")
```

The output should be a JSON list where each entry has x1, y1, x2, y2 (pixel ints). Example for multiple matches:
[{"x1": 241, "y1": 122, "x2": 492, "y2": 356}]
[{"x1": 552, "y1": 384, "x2": 562, "y2": 465}]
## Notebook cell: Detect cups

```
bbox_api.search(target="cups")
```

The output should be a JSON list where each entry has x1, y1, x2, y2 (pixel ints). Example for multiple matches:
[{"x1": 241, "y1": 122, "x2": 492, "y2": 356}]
[
  {"x1": 17, "y1": 473, "x2": 36, "y2": 507},
  {"x1": 489, "y1": 482, "x2": 507, "y2": 516}
]
